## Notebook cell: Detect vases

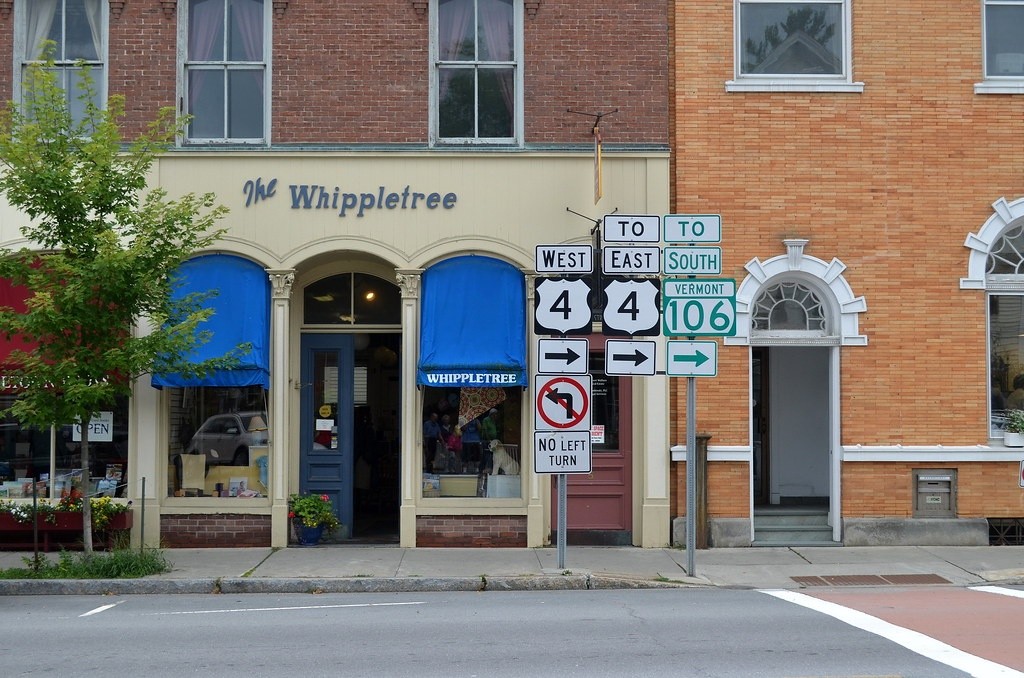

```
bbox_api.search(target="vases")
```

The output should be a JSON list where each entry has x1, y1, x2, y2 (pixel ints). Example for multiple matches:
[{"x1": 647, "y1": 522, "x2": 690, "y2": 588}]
[
  {"x1": 0, "y1": 509, "x2": 133, "y2": 553},
  {"x1": 292, "y1": 518, "x2": 323, "y2": 546}
]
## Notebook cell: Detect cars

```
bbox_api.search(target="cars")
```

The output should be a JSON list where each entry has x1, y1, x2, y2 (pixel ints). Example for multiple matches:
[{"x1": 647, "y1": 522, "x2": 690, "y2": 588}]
[{"x1": 186, "y1": 411, "x2": 268, "y2": 477}]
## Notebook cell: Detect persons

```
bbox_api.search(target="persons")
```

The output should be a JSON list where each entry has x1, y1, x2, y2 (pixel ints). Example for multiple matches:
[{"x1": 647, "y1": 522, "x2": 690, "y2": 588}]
[{"x1": 423, "y1": 408, "x2": 498, "y2": 473}]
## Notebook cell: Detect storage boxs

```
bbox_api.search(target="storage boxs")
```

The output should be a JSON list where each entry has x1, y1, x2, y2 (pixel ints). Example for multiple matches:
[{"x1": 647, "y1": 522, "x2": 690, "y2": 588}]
[
  {"x1": 439, "y1": 475, "x2": 479, "y2": 496},
  {"x1": 0, "y1": 464, "x2": 123, "y2": 498}
]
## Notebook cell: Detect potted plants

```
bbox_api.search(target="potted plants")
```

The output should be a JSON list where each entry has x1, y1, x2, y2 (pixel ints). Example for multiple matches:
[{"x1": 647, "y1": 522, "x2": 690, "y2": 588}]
[{"x1": 1004, "y1": 409, "x2": 1024, "y2": 446}]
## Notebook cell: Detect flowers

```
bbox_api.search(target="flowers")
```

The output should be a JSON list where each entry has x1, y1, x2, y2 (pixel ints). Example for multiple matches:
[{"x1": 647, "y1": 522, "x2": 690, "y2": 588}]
[
  {"x1": 0, "y1": 496, "x2": 129, "y2": 529},
  {"x1": 288, "y1": 493, "x2": 342, "y2": 532}
]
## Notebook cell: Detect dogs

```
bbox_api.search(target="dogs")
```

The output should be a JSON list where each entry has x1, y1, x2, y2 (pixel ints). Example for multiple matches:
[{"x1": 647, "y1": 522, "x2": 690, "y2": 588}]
[{"x1": 488, "y1": 439, "x2": 520, "y2": 475}]
